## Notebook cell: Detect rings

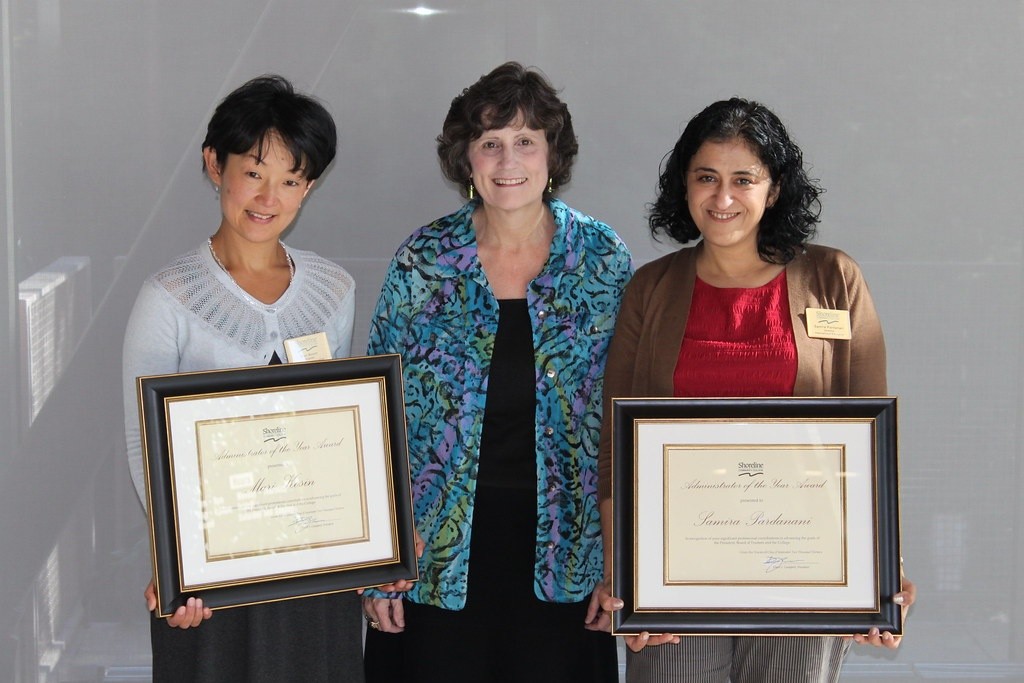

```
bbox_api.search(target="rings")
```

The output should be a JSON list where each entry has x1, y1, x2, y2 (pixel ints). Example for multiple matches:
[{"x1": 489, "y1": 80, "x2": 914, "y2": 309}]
[
  {"x1": 370, "y1": 621, "x2": 380, "y2": 629},
  {"x1": 365, "y1": 613, "x2": 372, "y2": 623}
]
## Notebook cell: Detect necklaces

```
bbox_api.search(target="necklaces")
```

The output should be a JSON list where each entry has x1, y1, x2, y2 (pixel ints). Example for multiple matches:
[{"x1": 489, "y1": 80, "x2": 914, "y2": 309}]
[{"x1": 207, "y1": 236, "x2": 294, "y2": 311}]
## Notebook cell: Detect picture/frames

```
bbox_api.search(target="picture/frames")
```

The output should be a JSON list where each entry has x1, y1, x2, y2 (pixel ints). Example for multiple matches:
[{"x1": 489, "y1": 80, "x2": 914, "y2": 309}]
[
  {"x1": 612, "y1": 396, "x2": 904, "y2": 637},
  {"x1": 137, "y1": 350, "x2": 419, "y2": 618}
]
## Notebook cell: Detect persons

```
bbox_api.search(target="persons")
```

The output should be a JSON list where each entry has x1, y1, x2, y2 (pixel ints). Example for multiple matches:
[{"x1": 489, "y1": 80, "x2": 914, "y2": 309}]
[
  {"x1": 364, "y1": 61, "x2": 636, "y2": 683},
  {"x1": 122, "y1": 72, "x2": 425, "y2": 683},
  {"x1": 599, "y1": 97, "x2": 914, "y2": 683}
]
